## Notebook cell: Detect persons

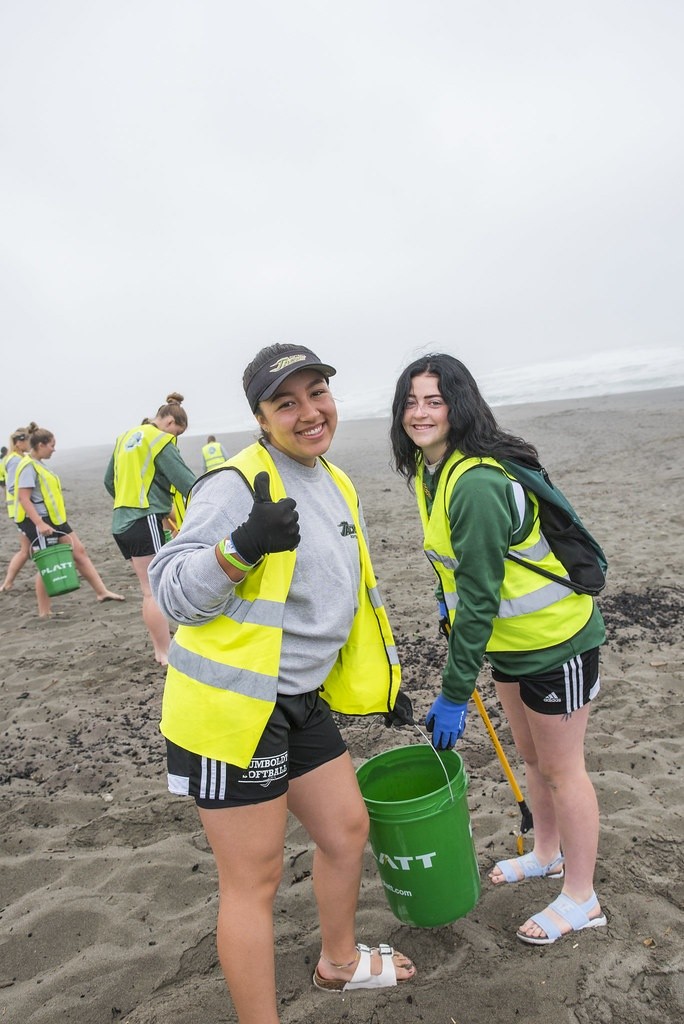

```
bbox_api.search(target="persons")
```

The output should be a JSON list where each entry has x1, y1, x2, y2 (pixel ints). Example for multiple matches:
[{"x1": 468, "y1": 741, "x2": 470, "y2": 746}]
[
  {"x1": 202, "y1": 436, "x2": 229, "y2": 472},
  {"x1": 0, "y1": 428, "x2": 33, "y2": 591},
  {"x1": 15, "y1": 420, "x2": 125, "y2": 617},
  {"x1": 106, "y1": 392, "x2": 198, "y2": 668},
  {"x1": 148, "y1": 344, "x2": 417, "y2": 1024},
  {"x1": 389, "y1": 356, "x2": 607, "y2": 947}
]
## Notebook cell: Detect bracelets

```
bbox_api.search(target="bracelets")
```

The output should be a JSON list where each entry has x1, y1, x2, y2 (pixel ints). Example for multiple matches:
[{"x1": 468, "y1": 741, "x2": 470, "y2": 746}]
[{"x1": 217, "y1": 539, "x2": 254, "y2": 571}]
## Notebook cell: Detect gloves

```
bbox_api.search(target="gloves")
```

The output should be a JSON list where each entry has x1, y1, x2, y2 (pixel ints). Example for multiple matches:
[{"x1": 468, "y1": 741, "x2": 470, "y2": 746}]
[
  {"x1": 424, "y1": 694, "x2": 467, "y2": 752},
  {"x1": 440, "y1": 604, "x2": 447, "y2": 617},
  {"x1": 383, "y1": 690, "x2": 414, "y2": 727},
  {"x1": 231, "y1": 471, "x2": 301, "y2": 566}
]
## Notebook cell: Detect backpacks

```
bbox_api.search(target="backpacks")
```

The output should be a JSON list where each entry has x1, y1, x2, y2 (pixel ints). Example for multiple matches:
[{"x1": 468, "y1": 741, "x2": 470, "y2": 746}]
[{"x1": 443, "y1": 453, "x2": 608, "y2": 595}]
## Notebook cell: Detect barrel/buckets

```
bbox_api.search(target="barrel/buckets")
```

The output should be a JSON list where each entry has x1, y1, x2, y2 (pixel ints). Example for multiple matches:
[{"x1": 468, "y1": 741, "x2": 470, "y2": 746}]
[
  {"x1": 354, "y1": 722, "x2": 480, "y2": 929},
  {"x1": 162, "y1": 529, "x2": 173, "y2": 542},
  {"x1": 28, "y1": 530, "x2": 80, "y2": 596}
]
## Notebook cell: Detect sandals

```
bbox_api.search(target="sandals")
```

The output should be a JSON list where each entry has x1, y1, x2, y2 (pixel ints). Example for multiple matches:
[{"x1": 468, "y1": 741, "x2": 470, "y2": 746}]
[
  {"x1": 488, "y1": 849, "x2": 564, "y2": 886},
  {"x1": 516, "y1": 889, "x2": 608, "y2": 945}
]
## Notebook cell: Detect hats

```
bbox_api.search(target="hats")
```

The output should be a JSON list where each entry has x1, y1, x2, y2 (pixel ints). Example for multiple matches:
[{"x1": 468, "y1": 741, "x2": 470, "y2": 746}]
[{"x1": 241, "y1": 342, "x2": 336, "y2": 414}]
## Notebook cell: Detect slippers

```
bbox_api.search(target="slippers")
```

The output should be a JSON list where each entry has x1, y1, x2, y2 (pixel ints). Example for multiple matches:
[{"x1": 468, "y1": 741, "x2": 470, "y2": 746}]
[{"x1": 313, "y1": 943, "x2": 417, "y2": 992}]
